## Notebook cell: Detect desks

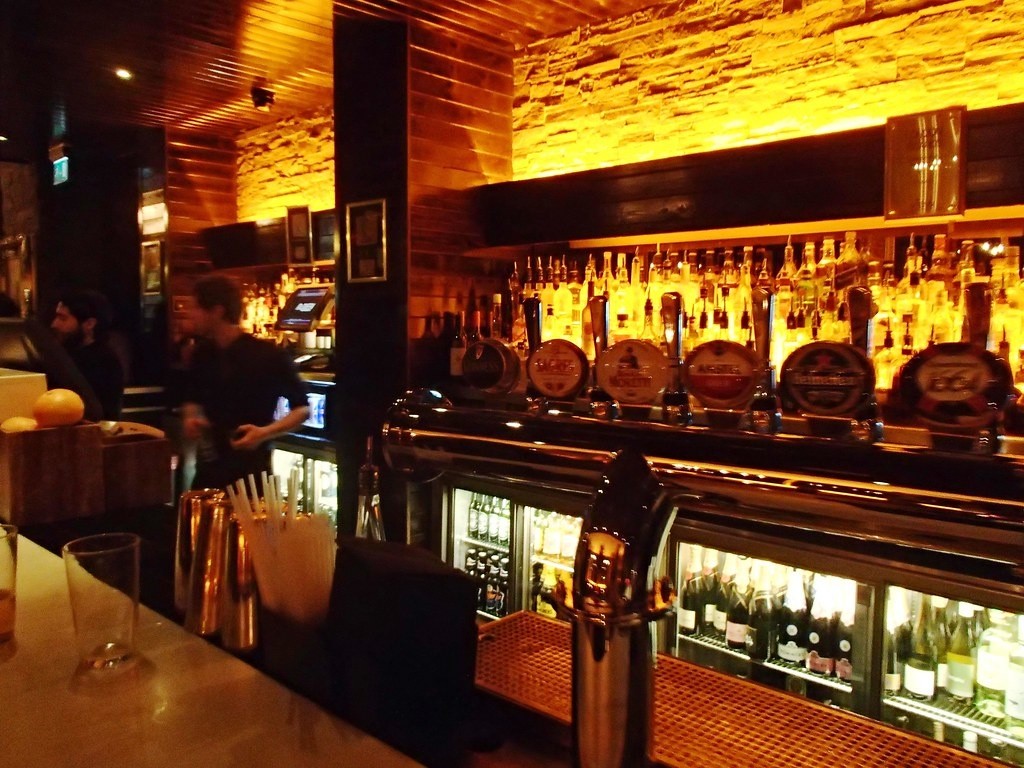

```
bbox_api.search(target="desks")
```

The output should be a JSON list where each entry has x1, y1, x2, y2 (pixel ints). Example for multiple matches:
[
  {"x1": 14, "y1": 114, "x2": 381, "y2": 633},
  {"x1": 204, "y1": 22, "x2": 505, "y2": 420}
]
[
  {"x1": 0, "y1": 534, "x2": 430, "y2": 768},
  {"x1": 0, "y1": 419, "x2": 174, "y2": 529}
]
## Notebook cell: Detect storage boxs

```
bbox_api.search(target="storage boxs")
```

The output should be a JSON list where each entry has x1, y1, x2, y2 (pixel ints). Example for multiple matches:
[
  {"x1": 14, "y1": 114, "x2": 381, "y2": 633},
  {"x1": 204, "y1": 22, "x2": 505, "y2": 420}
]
[{"x1": 0, "y1": 368, "x2": 47, "y2": 431}]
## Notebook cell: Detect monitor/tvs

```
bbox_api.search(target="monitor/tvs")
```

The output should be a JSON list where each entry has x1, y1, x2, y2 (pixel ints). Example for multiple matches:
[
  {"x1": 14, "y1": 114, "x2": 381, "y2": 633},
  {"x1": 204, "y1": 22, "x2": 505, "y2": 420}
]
[
  {"x1": 0, "y1": 317, "x2": 103, "y2": 422},
  {"x1": 274, "y1": 287, "x2": 332, "y2": 332}
]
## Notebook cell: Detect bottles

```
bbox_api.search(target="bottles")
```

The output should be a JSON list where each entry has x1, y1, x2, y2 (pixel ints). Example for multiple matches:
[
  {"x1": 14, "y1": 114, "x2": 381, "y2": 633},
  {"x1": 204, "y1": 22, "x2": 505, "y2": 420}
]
[
  {"x1": 466, "y1": 547, "x2": 510, "y2": 621},
  {"x1": 468, "y1": 493, "x2": 510, "y2": 546},
  {"x1": 891, "y1": 715, "x2": 998, "y2": 754},
  {"x1": 356, "y1": 437, "x2": 386, "y2": 540},
  {"x1": 508, "y1": 231, "x2": 1024, "y2": 390},
  {"x1": 881, "y1": 594, "x2": 1024, "y2": 725},
  {"x1": 680, "y1": 544, "x2": 854, "y2": 682},
  {"x1": 435, "y1": 293, "x2": 539, "y2": 393},
  {"x1": 531, "y1": 509, "x2": 582, "y2": 565},
  {"x1": 530, "y1": 563, "x2": 573, "y2": 618}
]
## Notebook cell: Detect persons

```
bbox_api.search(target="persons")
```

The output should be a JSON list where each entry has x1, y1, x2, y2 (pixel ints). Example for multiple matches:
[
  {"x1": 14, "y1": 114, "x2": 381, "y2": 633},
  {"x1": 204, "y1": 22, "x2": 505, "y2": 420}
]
[
  {"x1": 32, "y1": 284, "x2": 122, "y2": 421},
  {"x1": 159, "y1": 275, "x2": 311, "y2": 500}
]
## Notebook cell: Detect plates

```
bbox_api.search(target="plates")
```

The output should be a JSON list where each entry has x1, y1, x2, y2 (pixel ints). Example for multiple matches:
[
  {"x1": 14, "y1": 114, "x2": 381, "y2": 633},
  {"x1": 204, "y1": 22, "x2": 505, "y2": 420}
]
[{"x1": 98, "y1": 421, "x2": 164, "y2": 440}]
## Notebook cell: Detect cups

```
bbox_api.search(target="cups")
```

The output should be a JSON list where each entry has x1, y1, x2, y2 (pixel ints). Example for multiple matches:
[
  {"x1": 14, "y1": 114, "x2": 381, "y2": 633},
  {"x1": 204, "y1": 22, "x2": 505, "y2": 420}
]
[
  {"x1": 0, "y1": 524, "x2": 18, "y2": 658},
  {"x1": 62, "y1": 533, "x2": 140, "y2": 677},
  {"x1": 303, "y1": 329, "x2": 331, "y2": 349},
  {"x1": 174, "y1": 489, "x2": 335, "y2": 650}
]
